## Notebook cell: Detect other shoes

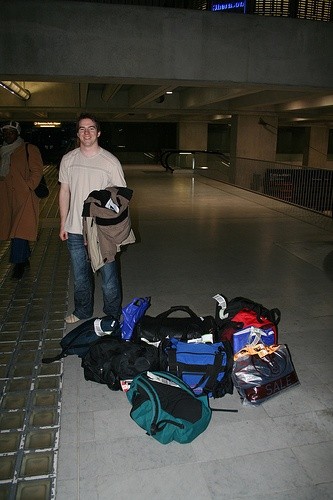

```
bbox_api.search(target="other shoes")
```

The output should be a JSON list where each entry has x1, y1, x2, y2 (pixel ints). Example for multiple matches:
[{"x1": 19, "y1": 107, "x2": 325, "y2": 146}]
[
  {"x1": 12, "y1": 263, "x2": 24, "y2": 280},
  {"x1": 24, "y1": 258, "x2": 30, "y2": 271},
  {"x1": 64, "y1": 314, "x2": 80, "y2": 324}
]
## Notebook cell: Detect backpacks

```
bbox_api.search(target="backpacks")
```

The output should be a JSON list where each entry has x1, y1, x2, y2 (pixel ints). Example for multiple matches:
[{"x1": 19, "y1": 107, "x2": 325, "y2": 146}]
[
  {"x1": 126, "y1": 370, "x2": 212, "y2": 444},
  {"x1": 42, "y1": 314, "x2": 120, "y2": 364},
  {"x1": 81, "y1": 335, "x2": 160, "y2": 391}
]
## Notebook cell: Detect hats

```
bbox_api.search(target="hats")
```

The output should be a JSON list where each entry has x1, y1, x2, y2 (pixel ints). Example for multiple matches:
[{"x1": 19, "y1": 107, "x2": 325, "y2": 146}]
[{"x1": 0, "y1": 120, "x2": 21, "y2": 135}]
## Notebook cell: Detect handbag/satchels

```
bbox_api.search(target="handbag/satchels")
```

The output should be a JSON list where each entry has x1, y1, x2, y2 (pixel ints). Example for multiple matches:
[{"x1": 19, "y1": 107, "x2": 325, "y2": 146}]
[
  {"x1": 120, "y1": 297, "x2": 151, "y2": 340},
  {"x1": 158, "y1": 337, "x2": 234, "y2": 399},
  {"x1": 34, "y1": 175, "x2": 49, "y2": 199},
  {"x1": 130, "y1": 306, "x2": 216, "y2": 344},
  {"x1": 212, "y1": 293, "x2": 301, "y2": 407}
]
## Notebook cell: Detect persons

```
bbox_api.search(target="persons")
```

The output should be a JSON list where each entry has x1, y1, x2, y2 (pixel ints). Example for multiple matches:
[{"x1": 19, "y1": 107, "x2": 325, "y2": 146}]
[
  {"x1": 0, "y1": 120, "x2": 50, "y2": 282},
  {"x1": 59, "y1": 113, "x2": 136, "y2": 324}
]
